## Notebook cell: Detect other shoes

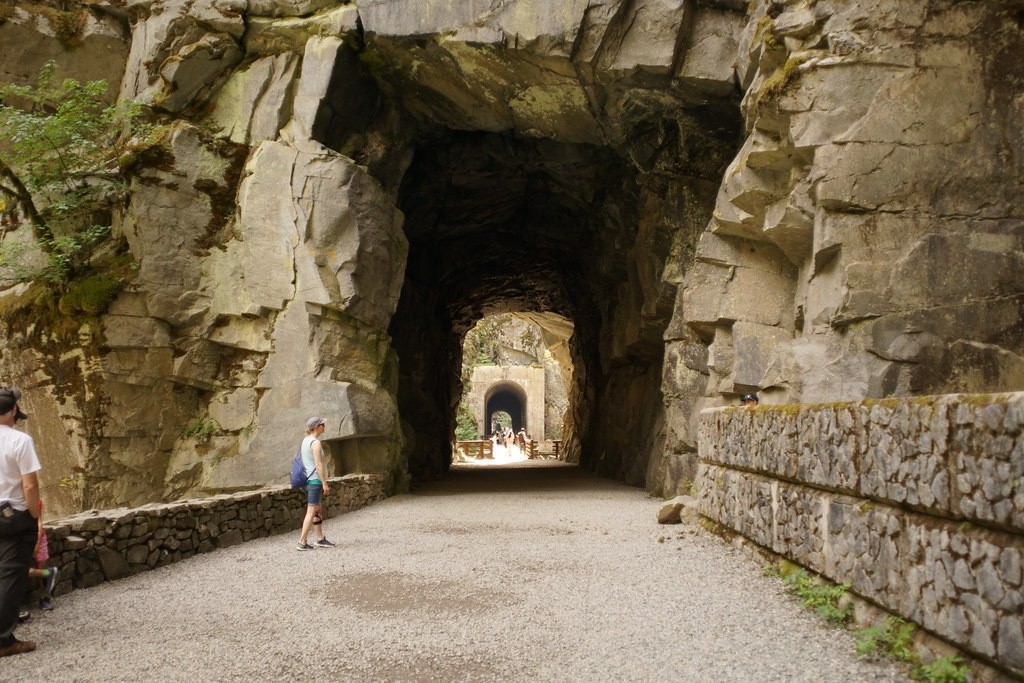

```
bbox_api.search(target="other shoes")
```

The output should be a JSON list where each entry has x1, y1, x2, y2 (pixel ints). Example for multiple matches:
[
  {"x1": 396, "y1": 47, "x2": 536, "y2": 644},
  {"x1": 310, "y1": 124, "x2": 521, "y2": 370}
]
[
  {"x1": 297, "y1": 543, "x2": 314, "y2": 551},
  {"x1": 18, "y1": 611, "x2": 30, "y2": 621},
  {"x1": 43, "y1": 567, "x2": 57, "y2": 592},
  {"x1": 0, "y1": 640, "x2": 35, "y2": 657},
  {"x1": 314, "y1": 539, "x2": 335, "y2": 547},
  {"x1": 37, "y1": 597, "x2": 52, "y2": 609}
]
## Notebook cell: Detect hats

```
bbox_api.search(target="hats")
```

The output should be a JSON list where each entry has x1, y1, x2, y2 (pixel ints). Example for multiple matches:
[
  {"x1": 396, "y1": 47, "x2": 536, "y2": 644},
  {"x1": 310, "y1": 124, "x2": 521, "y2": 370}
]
[
  {"x1": 307, "y1": 417, "x2": 327, "y2": 429},
  {"x1": 741, "y1": 394, "x2": 758, "y2": 401},
  {"x1": 0, "y1": 386, "x2": 20, "y2": 414},
  {"x1": 14, "y1": 405, "x2": 27, "y2": 419}
]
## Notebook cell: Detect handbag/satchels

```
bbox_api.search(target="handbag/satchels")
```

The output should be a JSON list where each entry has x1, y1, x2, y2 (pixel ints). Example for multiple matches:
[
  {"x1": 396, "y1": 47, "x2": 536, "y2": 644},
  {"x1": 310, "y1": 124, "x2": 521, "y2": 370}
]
[{"x1": 290, "y1": 455, "x2": 306, "y2": 488}]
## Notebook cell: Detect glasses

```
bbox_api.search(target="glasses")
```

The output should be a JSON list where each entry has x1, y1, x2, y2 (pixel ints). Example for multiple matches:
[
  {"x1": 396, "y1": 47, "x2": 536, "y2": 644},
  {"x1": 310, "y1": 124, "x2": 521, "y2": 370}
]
[
  {"x1": 320, "y1": 424, "x2": 325, "y2": 426},
  {"x1": 745, "y1": 395, "x2": 753, "y2": 400},
  {"x1": 0, "y1": 387, "x2": 14, "y2": 393},
  {"x1": 13, "y1": 417, "x2": 18, "y2": 421}
]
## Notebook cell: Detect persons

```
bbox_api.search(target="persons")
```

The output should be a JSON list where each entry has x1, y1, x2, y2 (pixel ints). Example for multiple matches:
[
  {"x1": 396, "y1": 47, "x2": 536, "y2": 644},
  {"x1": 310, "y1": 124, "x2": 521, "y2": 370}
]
[
  {"x1": 296, "y1": 417, "x2": 337, "y2": 551},
  {"x1": 494, "y1": 426, "x2": 527, "y2": 456},
  {"x1": 0, "y1": 385, "x2": 43, "y2": 659},
  {"x1": 15, "y1": 500, "x2": 58, "y2": 620}
]
